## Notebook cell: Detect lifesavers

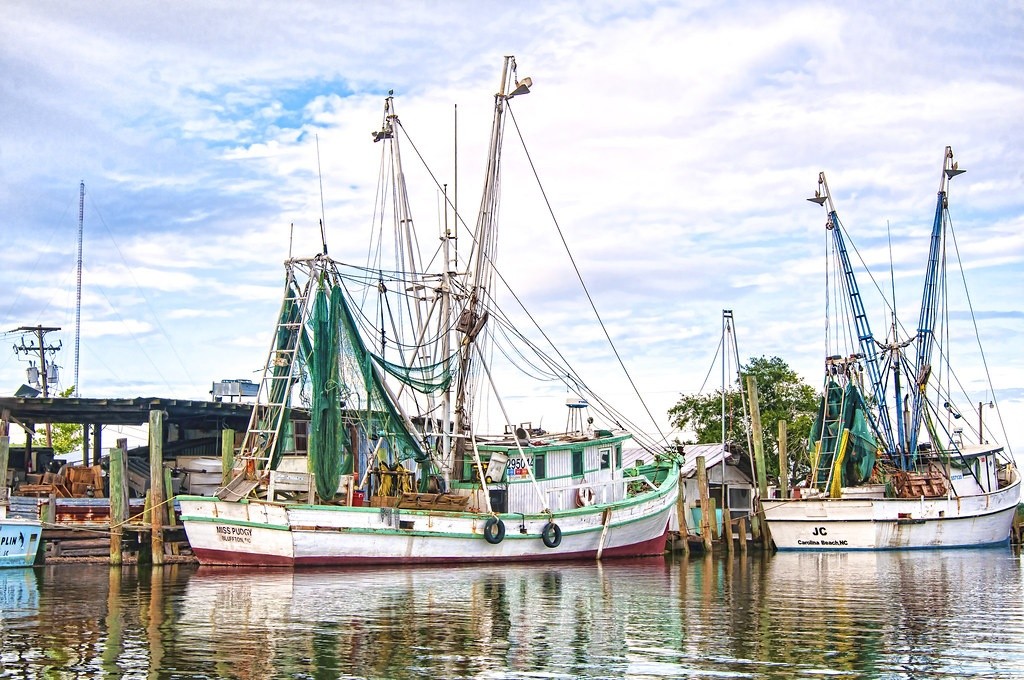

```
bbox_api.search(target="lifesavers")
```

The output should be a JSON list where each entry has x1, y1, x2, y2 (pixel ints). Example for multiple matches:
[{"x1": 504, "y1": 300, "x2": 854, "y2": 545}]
[{"x1": 579, "y1": 487, "x2": 596, "y2": 507}]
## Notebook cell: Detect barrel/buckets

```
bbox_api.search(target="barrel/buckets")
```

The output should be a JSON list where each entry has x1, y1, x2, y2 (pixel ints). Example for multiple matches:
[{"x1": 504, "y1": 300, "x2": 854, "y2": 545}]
[{"x1": 353, "y1": 489, "x2": 365, "y2": 506}]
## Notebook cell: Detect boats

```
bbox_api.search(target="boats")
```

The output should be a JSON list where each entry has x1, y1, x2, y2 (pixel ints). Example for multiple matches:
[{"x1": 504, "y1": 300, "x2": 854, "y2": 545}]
[
  {"x1": 0, "y1": 518, "x2": 43, "y2": 568},
  {"x1": 178, "y1": 54, "x2": 685, "y2": 567},
  {"x1": 761, "y1": 143, "x2": 1021, "y2": 551}
]
